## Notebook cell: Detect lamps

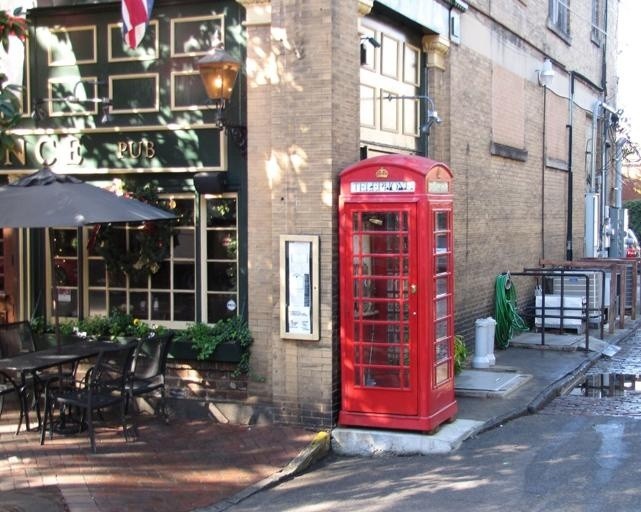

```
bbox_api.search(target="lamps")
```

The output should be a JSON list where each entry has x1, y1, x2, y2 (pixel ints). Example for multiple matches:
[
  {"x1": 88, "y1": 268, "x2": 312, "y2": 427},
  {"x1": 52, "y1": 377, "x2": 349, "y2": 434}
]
[
  {"x1": 386, "y1": 95, "x2": 444, "y2": 135},
  {"x1": 197, "y1": 44, "x2": 247, "y2": 157}
]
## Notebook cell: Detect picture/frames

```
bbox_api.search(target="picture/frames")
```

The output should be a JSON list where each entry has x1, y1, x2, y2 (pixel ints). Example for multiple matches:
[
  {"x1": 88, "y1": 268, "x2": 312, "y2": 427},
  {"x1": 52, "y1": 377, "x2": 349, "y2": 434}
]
[{"x1": 278, "y1": 234, "x2": 320, "y2": 342}]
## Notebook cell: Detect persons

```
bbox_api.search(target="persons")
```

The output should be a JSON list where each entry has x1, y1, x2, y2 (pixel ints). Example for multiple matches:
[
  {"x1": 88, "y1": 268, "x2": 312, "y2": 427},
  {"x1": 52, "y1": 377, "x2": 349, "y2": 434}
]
[{"x1": 602, "y1": 218, "x2": 612, "y2": 258}]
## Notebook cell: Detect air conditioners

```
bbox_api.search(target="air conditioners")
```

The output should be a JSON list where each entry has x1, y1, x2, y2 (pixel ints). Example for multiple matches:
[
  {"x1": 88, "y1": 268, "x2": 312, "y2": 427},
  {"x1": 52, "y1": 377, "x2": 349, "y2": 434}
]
[
  {"x1": 625, "y1": 264, "x2": 634, "y2": 309},
  {"x1": 552, "y1": 270, "x2": 602, "y2": 317}
]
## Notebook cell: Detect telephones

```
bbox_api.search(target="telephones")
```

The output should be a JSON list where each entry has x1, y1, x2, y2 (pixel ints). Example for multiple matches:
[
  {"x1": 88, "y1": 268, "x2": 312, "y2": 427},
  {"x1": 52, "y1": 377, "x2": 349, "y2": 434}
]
[{"x1": 353, "y1": 263, "x2": 370, "y2": 313}]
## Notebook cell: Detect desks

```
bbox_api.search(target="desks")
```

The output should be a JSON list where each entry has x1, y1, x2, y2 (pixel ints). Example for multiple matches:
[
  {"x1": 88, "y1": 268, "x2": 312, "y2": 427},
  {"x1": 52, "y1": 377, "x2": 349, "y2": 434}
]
[{"x1": 0, "y1": 341, "x2": 134, "y2": 434}]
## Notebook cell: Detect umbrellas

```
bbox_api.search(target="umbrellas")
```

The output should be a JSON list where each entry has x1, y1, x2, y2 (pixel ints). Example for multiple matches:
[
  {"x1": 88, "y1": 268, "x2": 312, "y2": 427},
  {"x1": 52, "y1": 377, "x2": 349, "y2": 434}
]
[{"x1": 0, "y1": 163, "x2": 178, "y2": 426}]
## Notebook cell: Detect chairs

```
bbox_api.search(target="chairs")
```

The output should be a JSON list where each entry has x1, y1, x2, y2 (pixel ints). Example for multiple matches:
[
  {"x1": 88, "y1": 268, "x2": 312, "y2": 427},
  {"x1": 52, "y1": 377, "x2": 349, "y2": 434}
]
[
  {"x1": 40, "y1": 341, "x2": 138, "y2": 450},
  {"x1": 0, "y1": 322, "x2": 69, "y2": 435},
  {"x1": 99, "y1": 333, "x2": 175, "y2": 434},
  {"x1": 14, "y1": 342, "x2": 16, "y2": 344},
  {"x1": 0, "y1": 377, "x2": 28, "y2": 438}
]
